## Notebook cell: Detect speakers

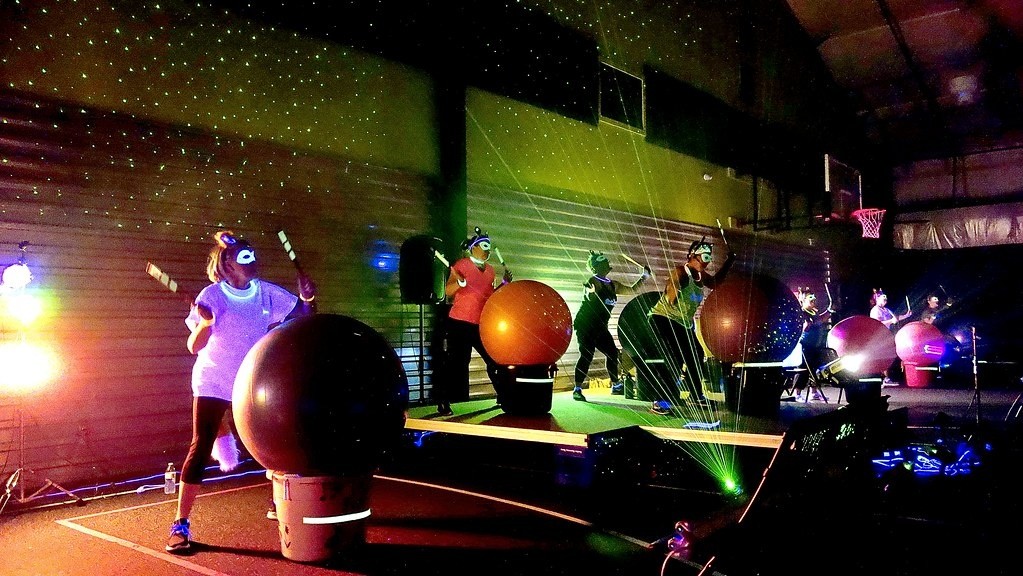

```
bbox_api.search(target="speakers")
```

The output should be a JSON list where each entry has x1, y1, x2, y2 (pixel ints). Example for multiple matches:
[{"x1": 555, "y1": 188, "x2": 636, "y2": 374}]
[{"x1": 399, "y1": 235, "x2": 447, "y2": 305}]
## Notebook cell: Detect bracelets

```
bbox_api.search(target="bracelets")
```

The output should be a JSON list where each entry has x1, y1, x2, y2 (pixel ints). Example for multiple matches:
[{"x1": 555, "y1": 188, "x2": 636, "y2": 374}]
[
  {"x1": 458, "y1": 279, "x2": 467, "y2": 288},
  {"x1": 502, "y1": 278, "x2": 509, "y2": 285},
  {"x1": 299, "y1": 293, "x2": 315, "y2": 302},
  {"x1": 826, "y1": 308, "x2": 835, "y2": 313},
  {"x1": 642, "y1": 273, "x2": 650, "y2": 277},
  {"x1": 588, "y1": 284, "x2": 596, "y2": 293},
  {"x1": 202, "y1": 312, "x2": 217, "y2": 325}
]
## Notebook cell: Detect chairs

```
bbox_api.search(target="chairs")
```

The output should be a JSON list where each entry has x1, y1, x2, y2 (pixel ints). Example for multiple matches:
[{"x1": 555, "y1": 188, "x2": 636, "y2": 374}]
[{"x1": 800, "y1": 343, "x2": 850, "y2": 404}]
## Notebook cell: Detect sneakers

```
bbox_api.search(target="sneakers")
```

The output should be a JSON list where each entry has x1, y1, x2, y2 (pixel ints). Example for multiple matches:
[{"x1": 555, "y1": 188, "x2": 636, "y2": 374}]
[
  {"x1": 685, "y1": 397, "x2": 718, "y2": 407},
  {"x1": 573, "y1": 387, "x2": 584, "y2": 399},
  {"x1": 266, "y1": 504, "x2": 278, "y2": 519},
  {"x1": 650, "y1": 407, "x2": 673, "y2": 414},
  {"x1": 796, "y1": 396, "x2": 806, "y2": 403},
  {"x1": 883, "y1": 379, "x2": 899, "y2": 386},
  {"x1": 165, "y1": 518, "x2": 189, "y2": 551},
  {"x1": 611, "y1": 384, "x2": 635, "y2": 394},
  {"x1": 811, "y1": 393, "x2": 829, "y2": 400}
]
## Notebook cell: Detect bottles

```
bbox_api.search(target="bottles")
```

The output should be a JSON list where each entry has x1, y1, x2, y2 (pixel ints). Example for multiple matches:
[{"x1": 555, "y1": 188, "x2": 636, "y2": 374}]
[
  {"x1": 625, "y1": 373, "x2": 633, "y2": 399},
  {"x1": 165, "y1": 462, "x2": 176, "y2": 494}
]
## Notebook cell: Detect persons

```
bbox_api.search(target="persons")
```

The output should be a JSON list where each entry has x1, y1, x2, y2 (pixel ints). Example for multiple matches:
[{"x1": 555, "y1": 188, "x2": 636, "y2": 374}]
[
  {"x1": 572, "y1": 235, "x2": 912, "y2": 415},
  {"x1": 156, "y1": 225, "x2": 321, "y2": 552},
  {"x1": 435, "y1": 226, "x2": 513, "y2": 416}
]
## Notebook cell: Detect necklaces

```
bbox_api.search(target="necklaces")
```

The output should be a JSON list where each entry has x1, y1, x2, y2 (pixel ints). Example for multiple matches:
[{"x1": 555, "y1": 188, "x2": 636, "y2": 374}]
[
  {"x1": 593, "y1": 274, "x2": 611, "y2": 282},
  {"x1": 802, "y1": 307, "x2": 816, "y2": 316},
  {"x1": 469, "y1": 256, "x2": 484, "y2": 265},
  {"x1": 220, "y1": 280, "x2": 256, "y2": 300},
  {"x1": 684, "y1": 262, "x2": 701, "y2": 282}
]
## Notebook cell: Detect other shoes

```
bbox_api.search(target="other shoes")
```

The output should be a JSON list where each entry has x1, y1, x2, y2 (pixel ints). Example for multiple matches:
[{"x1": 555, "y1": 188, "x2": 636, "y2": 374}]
[{"x1": 437, "y1": 402, "x2": 453, "y2": 415}]
